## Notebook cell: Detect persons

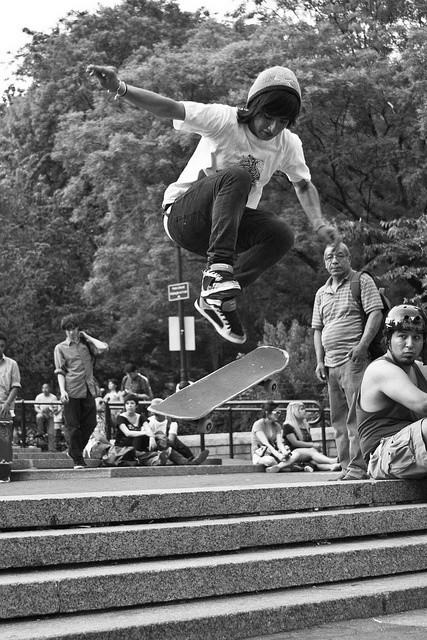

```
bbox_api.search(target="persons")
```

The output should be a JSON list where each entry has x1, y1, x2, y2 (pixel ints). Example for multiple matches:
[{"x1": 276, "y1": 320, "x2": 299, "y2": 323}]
[
  {"x1": 356, "y1": 303, "x2": 427, "y2": 480},
  {"x1": 53, "y1": 314, "x2": 110, "y2": 469},
  {"x1": 250, "y1": 400, "x2": 301, "y2": 473},
  {"x1": 140, "y1": 395, "x2": 210, "y2": 467},
  {"x1": 121, "y1": 363, "x2": 154, "y2": 401},
  {"x1": 82, "y1": 396, "x2": 138, "y2": 467},
  {"x1": 311, "y1": 242, "x2": 384, "y2": 480},
  {"x1": 0, "y1": 333, "x2": 22, "y2": 482},
  {"x1": 282, "y1": 401, "x2": 342, "y2": 473},
  {"x1": 99, "y1": 378, "x2": 121, "y2": 402},
  {"x1": 34, "y1": 383, "x2": 57, "y2": 438},
  {"x1": 86, "y1": 63, "x2": 342, "y2": 344},
  {"x1": 115, "y1": 392, "x2": 157, "y2": 465}
]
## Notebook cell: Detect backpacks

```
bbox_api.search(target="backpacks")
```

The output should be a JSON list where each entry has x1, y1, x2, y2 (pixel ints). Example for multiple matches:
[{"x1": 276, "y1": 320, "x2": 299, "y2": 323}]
[{"x1": 349, "y1": 268, "x2": 390, "y2": 352}]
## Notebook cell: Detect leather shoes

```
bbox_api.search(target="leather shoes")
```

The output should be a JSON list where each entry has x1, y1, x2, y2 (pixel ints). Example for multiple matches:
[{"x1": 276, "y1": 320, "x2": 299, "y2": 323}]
[
  {"x1": 331, "y1": 474, "x2": 346, "y2": 482},
  {"x1": 341, "y1": 474, "x2": 366, "y2": 480},
  {"x1": 190, "y1": 449, "x2": 210, "y2": 465}
]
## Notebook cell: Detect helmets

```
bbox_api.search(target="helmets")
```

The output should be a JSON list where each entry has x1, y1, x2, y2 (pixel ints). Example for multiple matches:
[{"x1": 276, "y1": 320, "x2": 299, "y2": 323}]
[{"x1": 385, "y1": 304, "x2": 425, "y2": 333}]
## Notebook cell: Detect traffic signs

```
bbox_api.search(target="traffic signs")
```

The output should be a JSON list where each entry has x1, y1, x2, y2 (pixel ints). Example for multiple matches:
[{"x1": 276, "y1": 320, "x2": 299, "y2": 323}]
[
  {"x1": 167, "y1": 316, "x2": 196, "y2": 352},
  {"x1": 167, "y1": 282, "x2": 191, "y2": 302}
]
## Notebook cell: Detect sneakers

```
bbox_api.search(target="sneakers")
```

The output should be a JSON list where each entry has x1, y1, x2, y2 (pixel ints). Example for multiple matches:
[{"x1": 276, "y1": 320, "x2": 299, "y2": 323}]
[
  {"x1": 73, "y1": 463, "x2": 86, "y2": 469},
  {"x1": 265, "y1": 465, "x2": 281, "y2": 473},
  {"x1": 200, "y1": 264, "x2": 243, "y2": 300},
  {"x1": 193, "y1": 296, "x2": 249, "y2": 344}
]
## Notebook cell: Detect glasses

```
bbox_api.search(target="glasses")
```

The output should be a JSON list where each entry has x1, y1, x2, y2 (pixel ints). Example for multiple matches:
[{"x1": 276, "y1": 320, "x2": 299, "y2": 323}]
[
  {"x1": 97, "y1": 401, "x2": 107, "y2": 405},
  {"x1": 269, "y1": 410, "x2": 283, "y2": 416}
]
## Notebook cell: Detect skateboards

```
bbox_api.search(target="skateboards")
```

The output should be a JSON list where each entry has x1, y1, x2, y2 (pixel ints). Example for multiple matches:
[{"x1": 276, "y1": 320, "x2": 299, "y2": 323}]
[{"x1": 147, "y1": 346, "x2": 288, "y2": 433}]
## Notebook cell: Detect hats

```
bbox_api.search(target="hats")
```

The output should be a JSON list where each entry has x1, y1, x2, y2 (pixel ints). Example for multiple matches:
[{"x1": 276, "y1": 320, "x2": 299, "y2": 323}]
[{"x1": 246, "y1": 78, "x2": 303, "y2": 107}]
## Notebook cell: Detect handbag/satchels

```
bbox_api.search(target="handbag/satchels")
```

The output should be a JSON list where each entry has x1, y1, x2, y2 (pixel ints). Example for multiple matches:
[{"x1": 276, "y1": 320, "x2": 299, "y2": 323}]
[{"x1": 102, "y1": 445, "x2": 137, "y2": 466}]
